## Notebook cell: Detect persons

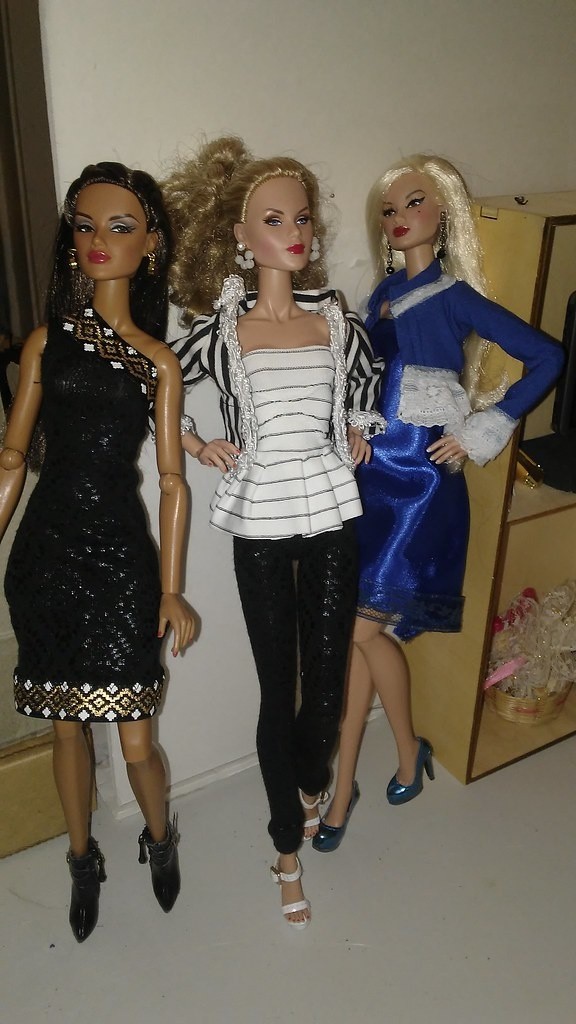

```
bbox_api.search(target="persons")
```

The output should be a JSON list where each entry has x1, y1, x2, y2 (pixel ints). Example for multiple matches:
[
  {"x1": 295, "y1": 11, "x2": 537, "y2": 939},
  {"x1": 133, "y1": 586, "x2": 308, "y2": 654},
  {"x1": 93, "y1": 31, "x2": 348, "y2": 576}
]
[
  {"x1": 0, "y1": 162, "x2": 195, "y2": 943},
  {"x1": 145, "y1": 136, "x2": 387, "y2": 930},
  {"x1": 311, "y1": 152, "x2": 571, "y2": 854}
]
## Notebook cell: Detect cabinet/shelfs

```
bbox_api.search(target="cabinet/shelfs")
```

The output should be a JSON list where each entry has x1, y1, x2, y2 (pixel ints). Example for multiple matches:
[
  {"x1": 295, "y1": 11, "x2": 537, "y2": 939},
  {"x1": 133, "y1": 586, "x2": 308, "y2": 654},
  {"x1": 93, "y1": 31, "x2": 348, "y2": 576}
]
[{"x1": 398, "y1": 190, "x2": 576, "y2": 786}]
